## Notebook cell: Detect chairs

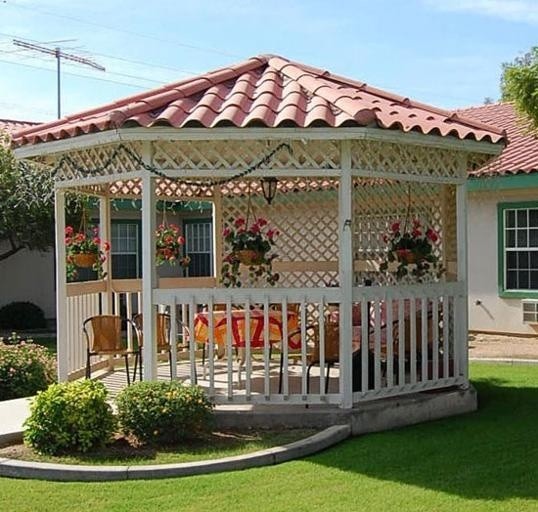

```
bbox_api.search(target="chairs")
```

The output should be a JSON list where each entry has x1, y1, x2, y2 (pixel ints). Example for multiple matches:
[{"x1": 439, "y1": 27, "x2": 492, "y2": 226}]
[
  {"x1": 130, "y1": 311, "x2": 199, "y2": 389},
  {"x1": 189, "y1": 302, "x2": 443, "y2": 409},
  {"x1": 82, "y1": 313, "x2": 143, "y2": 390}
]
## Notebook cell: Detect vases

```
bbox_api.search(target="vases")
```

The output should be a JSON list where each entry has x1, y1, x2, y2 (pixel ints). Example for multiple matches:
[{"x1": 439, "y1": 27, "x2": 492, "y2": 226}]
[
  {"x1": 235, "y1": 250, "x2": 256, "y2": 265},
  {"x1": 74, "y1": 254, "x2": 94, "y2": 268},
  {"x1": 396, "y1": 250, "x2": 414, "y2": 263}
]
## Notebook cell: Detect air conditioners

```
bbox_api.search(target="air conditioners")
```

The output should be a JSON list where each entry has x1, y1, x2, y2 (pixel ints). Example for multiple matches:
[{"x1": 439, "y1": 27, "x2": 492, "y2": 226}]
[{"x1": 520, "y1": 299, "x2": 538, "y2": 325}]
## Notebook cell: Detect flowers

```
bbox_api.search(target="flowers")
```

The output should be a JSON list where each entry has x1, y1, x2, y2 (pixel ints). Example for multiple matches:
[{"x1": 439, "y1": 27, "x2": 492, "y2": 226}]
[
  {"x1": 64, "y1": 226, "x2": 111, "y2": 281},
  {"x1": 219, "y1": 218, "x2": 279, "y2": 288},
  {"x1": 378, "y1": 219, "x2": 447, "y2": 279}
]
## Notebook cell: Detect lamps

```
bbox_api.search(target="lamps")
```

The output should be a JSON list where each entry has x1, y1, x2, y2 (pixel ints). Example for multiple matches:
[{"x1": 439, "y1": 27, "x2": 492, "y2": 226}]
[{"x1": 259, "y1": 140, "x2": 280, "y2": 205}]
